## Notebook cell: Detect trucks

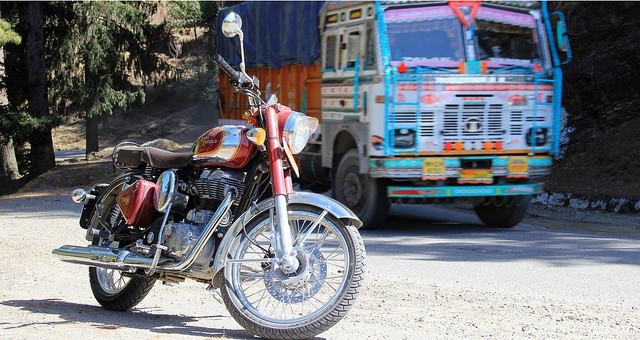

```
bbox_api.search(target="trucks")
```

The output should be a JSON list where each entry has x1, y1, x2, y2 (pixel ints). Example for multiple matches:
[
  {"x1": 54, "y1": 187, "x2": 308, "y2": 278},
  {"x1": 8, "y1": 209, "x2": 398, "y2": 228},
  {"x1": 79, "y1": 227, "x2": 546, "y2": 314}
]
[{"x1": 215, "y1": 1, "x2": 573, "y2": 229}]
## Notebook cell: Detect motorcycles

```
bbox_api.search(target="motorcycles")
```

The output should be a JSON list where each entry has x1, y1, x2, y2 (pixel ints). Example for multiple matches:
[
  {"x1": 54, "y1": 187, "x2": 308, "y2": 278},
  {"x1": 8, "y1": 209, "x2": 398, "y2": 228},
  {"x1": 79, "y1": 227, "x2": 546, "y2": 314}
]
[{"x1": 52, "y1": 12, "x2": 366, "y2": 339}]
